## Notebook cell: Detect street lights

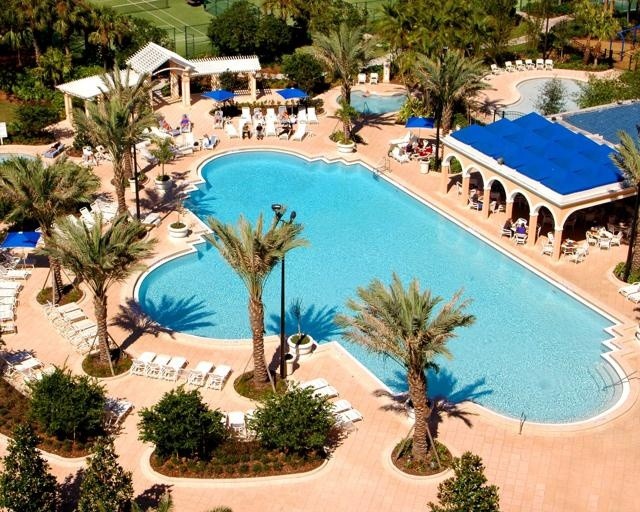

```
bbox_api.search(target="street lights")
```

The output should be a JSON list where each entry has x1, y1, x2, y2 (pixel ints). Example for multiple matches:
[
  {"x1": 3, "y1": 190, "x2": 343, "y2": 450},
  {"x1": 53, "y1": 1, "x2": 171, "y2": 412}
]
[
  {"x1": 128, "y1": 97, "x2": 140, "y2": 223},
  {"x1": 272, "y1": 204, "x2": 296, "y2": 377}
]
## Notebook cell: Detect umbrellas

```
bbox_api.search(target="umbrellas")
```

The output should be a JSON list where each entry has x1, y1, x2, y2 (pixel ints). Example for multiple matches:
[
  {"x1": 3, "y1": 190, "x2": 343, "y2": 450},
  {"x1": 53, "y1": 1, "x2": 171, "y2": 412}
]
[
  {"x1": 404, "y1": 115, "x2": 434, "y2": 137},
  {"x1": 0, "y1": 230, "x2": 43, "y2": 270},
  {"x1": 200, "y1": 88, "x2": 239, "y2": 111},
  {"x1": 275, "y1": 86, "x2": 310, "y2": 115}
]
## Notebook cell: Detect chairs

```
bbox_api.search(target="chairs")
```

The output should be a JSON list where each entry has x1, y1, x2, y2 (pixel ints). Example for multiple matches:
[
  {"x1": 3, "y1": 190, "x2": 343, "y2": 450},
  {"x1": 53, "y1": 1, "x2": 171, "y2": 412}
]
[
  {"x1": 390, "y1": 142, "x2": 437, "y2": 164},
  {"x1": 296, "y1": 378, "x2": 361, "y2": 430},
  {"x1": 357, "y1": 73, "x2": 366, "y2": 86},
  {"x1": 1, "y1": 254, "x2": 33, "y2": 336},
  {"x1": 102, "y1": 396, "x2": 130, "y2": 429},
  {"x1": 42, "y1": 299, "x2": 112, "y2": 356},
  {"x1": 43, "y1": 142, "x2": 65, "y2": 159},
  {"x1": 456, "y1": 181, "x2": 624, "y2": 264},
  {"x1": 370, "y1": 73, "x2": 378, "y2": 85},
  {"x1": 131, "y1": 352, "x2": 232, "y2": 391},
  {"x1": 490, "y1": 59, "x2": 552, "y2": 75},
  {"x1": 1, "y1": 348, "x2": 59, "y2": 387},
  {"x1": 34, "y1": 199, "x2": 119, "y2": 250},
  {"x1": 81, "y1": 107, "x2": 318, "y2": 165},
  {"x1": 218, "y1": 410, "x2": 261, "y2": 438}
]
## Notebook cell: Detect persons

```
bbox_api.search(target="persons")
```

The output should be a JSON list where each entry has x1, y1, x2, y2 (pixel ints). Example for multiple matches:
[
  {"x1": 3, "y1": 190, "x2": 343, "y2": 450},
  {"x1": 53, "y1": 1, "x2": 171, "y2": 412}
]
[
  {"x1": 503, "y1": 217, "x2": 515, "y2": 239},
  {"x1": 215, "y1": 111, "x2": 222, "y2": 126},
  {"x1": 515, "y1": 223, "x2": 527, "y2": 233},
  {"x1": 98, "y1": 147, "x2": 111, "y2": 160},
  {"x1": 242, "y1": 123, "x2": 251, "y2": 140},
  {"x1": 162, "y1": 119, "x2": 172, "y2": 130},
  {"x1": 181, "y1": 115, "x2": 190, "y2": 129},
  {"x1": 257, "y1": 110, "x2": 263, "y2": 120},
  {"x1": 255, "y1": 122, "x2": 264, "y2": 140},
  {"x1": 405, "y1": 138, "x2": 433, "y2": 161},
  {"x1": 202, "y1": 132, "x2": 211, "y2": 147},
  {"x1": 0, "y1": 247, "x2": 24, "y2": 270},
  {"x1": 471, "y1": 189, "x2": 483, "y2": 211},
  {"x1": 280, "y1": 110, "x2": 289, "y2": 120},
  {"x1": 45, "y1": 141, "x2": 62, "y2": 154}
]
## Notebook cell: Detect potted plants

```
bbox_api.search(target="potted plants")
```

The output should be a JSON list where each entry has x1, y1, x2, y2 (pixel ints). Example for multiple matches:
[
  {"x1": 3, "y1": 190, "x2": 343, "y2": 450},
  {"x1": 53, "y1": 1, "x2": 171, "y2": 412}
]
[
  {"x1": 278, "y1": 294, "x2": 314, "y2": 375},
  {"x1": 150, "y1": 135, "x2": 176, "y2": 190},
  {"x1": 420, "y1": 157, "x2": 430, "y2": 174},
  {"x1": 167, "y1": 184, "x2": 190, "y2": 239},
  {"x1": 335, "y1": 98, "x2": 359, "y2": 152},
  {"x1": 128, "y1": 177, "x2": 139, "y2": 192}
]
